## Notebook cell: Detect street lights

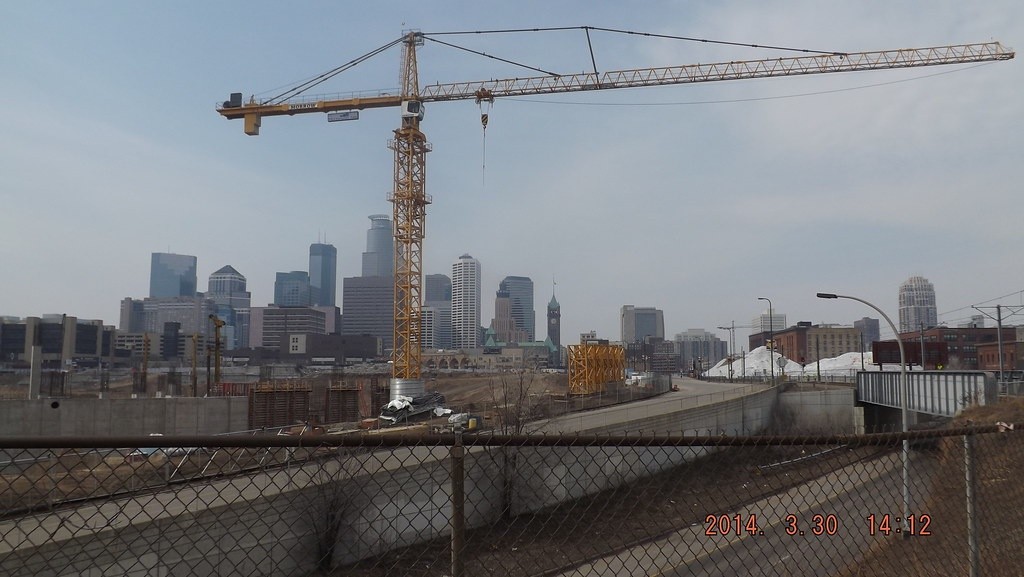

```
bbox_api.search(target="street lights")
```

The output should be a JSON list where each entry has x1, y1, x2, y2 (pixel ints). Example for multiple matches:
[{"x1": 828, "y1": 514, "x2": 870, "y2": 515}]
[
  {"x1": 717, "y1": 326, "x2": 732, "y2": 376},
  {"x1": 757, "y1": 296, "x2": 774, "y2": 379},
  {"x1": 813, "y1": 288, "x2": 915, "y2": 541}
]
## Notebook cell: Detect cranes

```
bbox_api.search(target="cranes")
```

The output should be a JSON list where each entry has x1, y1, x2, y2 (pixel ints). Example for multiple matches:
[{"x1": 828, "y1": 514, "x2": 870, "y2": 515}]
[{"x1": 213, "y1": 26, "x2": 1018, "y2": 381}]
